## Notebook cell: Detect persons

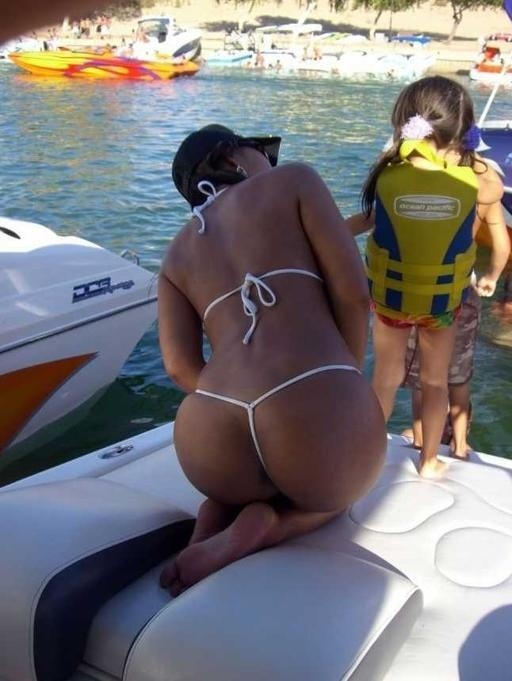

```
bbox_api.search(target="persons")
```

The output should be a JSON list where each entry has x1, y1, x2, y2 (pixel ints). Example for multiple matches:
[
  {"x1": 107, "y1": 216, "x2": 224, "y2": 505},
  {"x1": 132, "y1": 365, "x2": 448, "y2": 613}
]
[
  {"x1": 365, "y1": 74, "x2": 504, "y2": 474},
  {"x1": 155, "y1": 122, "x2": 389, "y2": 596},
  {"x1": 345, "y1": 153, "x2": 512, "y2": 459},
  {"x1": 10, "y1": 11, "x2": 324, "y2": 74}
]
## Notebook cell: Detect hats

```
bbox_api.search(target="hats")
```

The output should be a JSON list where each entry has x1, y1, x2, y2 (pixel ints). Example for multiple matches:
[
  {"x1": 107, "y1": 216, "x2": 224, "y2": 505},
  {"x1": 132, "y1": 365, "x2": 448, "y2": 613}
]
[{"x1": 172, "y1": 123, "x2": 281, "y2": 200}]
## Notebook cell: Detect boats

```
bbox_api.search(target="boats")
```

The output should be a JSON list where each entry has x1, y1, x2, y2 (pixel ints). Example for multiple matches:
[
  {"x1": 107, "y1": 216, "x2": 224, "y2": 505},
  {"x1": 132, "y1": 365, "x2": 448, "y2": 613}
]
[
  {"x1": 4, "y1": 30, "x2": 212, "y2": 84},
  {"x1": 205, "y1": 22, "x2": 440, "y2": 78},
  {"x1": 470, "y1": 111, "x2": 512, "y2": 248},
  {"x1": 0, "y1": 213, "x2": 166, "y2": 458},
  {"x1": 468, "y1": 32, "x2": 512, "y2": 85}
]
